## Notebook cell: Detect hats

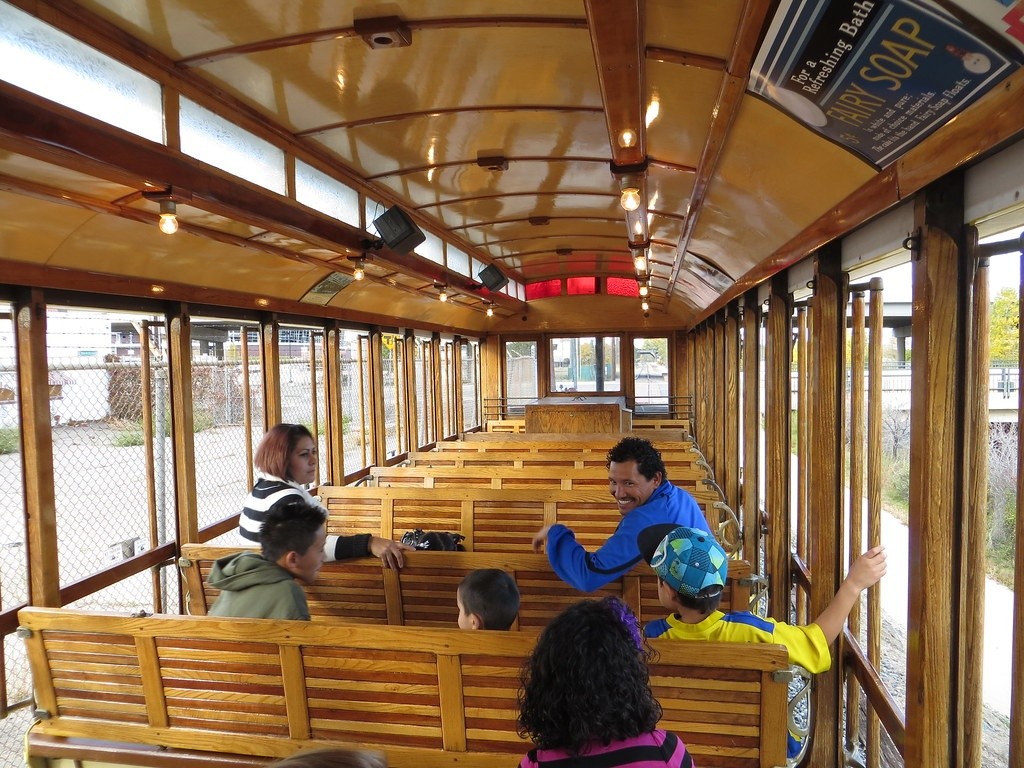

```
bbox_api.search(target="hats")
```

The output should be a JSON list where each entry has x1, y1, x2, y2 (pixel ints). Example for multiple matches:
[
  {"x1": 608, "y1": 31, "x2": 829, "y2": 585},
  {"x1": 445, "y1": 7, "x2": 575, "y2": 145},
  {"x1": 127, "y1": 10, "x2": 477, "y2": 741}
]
[{"x1": 636, "y1": 523, "x2": 728, "y2": 599}]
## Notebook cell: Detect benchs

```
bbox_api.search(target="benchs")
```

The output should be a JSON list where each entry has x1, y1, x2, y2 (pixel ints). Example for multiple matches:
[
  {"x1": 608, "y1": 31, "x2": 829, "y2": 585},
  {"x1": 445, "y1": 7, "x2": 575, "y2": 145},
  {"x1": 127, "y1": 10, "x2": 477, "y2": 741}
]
[{"x1": 18, "y1": 421, "x2": 795, "y2": 768}]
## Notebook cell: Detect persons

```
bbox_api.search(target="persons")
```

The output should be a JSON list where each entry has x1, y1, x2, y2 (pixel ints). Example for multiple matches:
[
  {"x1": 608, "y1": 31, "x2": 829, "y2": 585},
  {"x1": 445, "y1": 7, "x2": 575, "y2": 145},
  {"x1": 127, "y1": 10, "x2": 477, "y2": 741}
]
[
  {"x1": 205, "y1": 502, "x2": 328, "y2": 622},
  {"x1": 456, "y1": 568, "x2": 521, "y2": 631},
  {"x1": 636, "y1": 522, "x2": 888, "y2": 768},
  {"x1": 238, "y1": 423, "x2": 417, "y2": 570},
  {"x1": 515, "y1": 595, "x2": 697, "y2": 768},
  {"x1": 532, "y1": 437, "x2": 716, "y2": 595},
  {"x1": 260, "y1": 746, "x2": 388, "y2": 767}
]
